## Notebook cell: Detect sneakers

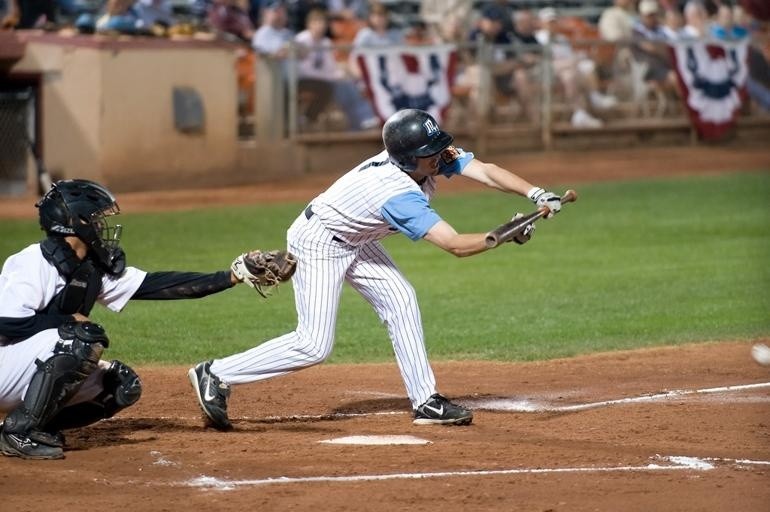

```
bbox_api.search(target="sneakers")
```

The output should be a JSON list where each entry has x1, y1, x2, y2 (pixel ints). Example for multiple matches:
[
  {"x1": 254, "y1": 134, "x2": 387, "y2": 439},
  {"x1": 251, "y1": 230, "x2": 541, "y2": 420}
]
[
  {"x1": 185, "y1": 356, "x2": 237, "y2": 431},
  {"x1": 410, "y1": 392, "x2": 477, "y2": 428},
  {"x1": 0, "y1": 423, "x2": 66, "y2": 461}
]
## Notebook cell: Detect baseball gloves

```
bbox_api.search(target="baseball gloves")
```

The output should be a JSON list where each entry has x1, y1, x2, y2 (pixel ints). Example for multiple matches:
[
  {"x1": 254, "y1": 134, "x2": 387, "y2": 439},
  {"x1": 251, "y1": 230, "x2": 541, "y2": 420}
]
[{"x1": 230, "y1": 250, "x2": 298, "y2": 297}]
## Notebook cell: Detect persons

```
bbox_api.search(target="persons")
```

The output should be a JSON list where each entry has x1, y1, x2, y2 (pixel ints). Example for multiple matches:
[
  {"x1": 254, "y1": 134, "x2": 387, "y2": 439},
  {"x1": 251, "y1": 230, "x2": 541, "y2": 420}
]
[
  {"x1": 0, "y1": 0, "x2": 770, "y2": 140},
  {"x1": 0, "y1": 178, "x2": 297, "y2": 460},
  {"x1": 188, "y1": 109, "x2": 562, "y2": 428}
]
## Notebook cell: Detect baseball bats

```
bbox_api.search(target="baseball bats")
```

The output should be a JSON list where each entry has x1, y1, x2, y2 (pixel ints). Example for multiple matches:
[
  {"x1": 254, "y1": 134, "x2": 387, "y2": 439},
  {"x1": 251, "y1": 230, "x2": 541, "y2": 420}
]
[{"x1": 484, "y1": 189, "x2": 577, "y2": 249}]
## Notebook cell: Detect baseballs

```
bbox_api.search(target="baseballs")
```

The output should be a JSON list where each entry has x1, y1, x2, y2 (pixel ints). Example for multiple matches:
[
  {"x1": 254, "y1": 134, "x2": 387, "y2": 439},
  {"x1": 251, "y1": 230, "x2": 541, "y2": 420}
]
[{"x1": 752, "y1": 343, "x2": 770, "y2": 366}]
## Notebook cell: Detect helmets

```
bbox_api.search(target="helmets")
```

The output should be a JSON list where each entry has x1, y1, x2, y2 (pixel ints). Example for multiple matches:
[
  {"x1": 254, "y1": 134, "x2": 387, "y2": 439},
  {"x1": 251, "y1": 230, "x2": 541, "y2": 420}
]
[
  {"x1": 381, "y1": 107, "x2": 456, "y2": 174},
  {"x1": 33, "y1": 177, "x2": 116, "y2": 268}
]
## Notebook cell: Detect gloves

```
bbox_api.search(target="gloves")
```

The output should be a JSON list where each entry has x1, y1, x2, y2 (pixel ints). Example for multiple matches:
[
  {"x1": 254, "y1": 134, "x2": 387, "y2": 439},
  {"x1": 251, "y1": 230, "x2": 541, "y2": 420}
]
[
  {"x1": 506, "y1": 211, "x2": 540, "y2": 247},
  {"x1": 524, "y1": 184, "x2": 564, "y2": 220}
]
